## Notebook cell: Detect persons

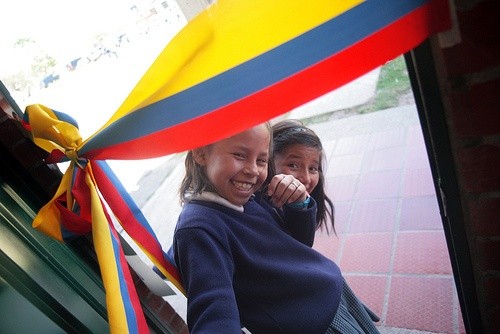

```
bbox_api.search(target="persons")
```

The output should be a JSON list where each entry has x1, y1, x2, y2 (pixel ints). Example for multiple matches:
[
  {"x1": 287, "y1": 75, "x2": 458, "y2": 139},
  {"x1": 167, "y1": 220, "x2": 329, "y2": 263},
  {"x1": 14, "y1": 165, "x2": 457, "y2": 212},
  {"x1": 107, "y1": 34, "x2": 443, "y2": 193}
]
[
  {"x1": 152, "y1": 122, "x2": 337, "y2": 279},
  {"x1": 173, "y1": 121, "x2": 380, "y2": 334}
]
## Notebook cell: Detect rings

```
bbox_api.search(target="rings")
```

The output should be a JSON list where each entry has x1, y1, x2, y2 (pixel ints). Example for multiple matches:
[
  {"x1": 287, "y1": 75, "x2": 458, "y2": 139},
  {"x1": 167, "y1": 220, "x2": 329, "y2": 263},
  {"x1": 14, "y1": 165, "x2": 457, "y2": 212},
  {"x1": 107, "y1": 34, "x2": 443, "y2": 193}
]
[{"x1": 291, "y1": 181, "x2": 298, "y2": 188}]
